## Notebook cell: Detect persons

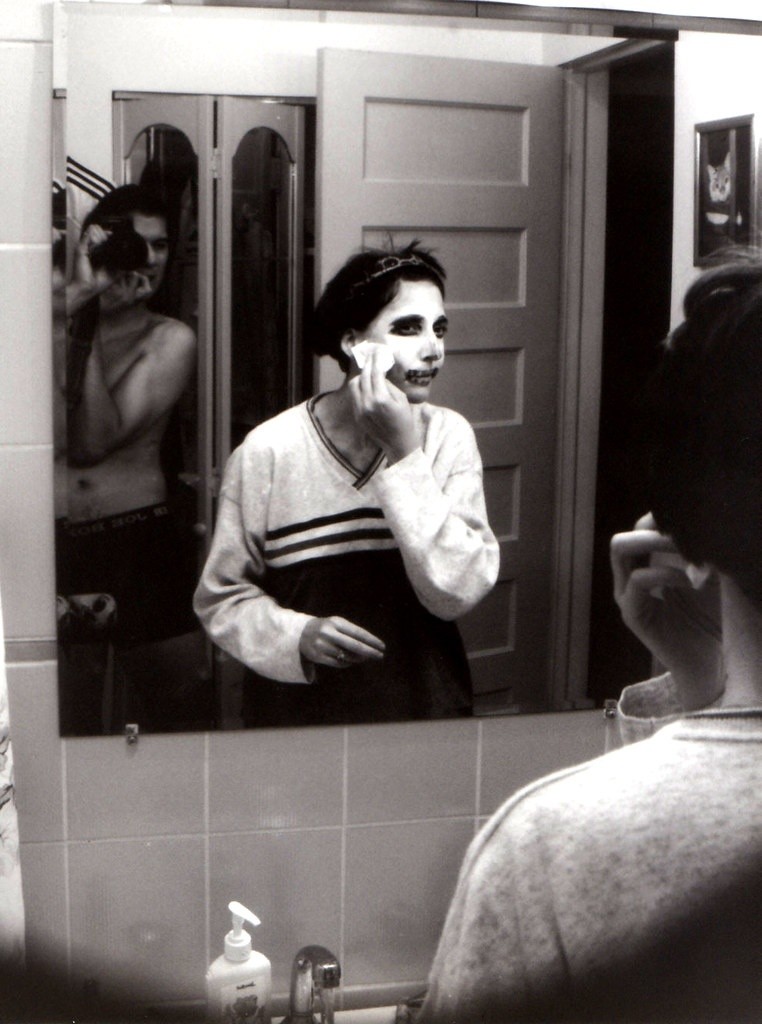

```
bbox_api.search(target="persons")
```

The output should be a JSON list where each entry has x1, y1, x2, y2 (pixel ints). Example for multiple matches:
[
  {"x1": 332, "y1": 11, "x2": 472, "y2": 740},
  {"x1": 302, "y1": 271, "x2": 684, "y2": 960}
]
[
  {"x1": 50, "y1": 182, "x2": 197, "y2": 738},
  {"x1": 189, "y1": 240, "x2": 501, "y2": 727},
  {"x1": 410, "y1": 260, "x2": 762, "y2": 1024}
]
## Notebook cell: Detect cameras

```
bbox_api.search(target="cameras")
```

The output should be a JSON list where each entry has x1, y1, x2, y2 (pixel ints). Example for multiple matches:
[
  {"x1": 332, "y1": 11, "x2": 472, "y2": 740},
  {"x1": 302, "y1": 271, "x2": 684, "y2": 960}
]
[{"x1": 89, "y1": 217, "x2": 148, "y2": 272}]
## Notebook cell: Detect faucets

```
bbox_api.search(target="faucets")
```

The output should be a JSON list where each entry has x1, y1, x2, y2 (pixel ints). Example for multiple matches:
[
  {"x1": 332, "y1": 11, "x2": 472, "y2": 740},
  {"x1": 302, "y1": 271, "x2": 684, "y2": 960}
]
[{"x1": 278, "y1": 944, "x2": 343, "y2": 1024}]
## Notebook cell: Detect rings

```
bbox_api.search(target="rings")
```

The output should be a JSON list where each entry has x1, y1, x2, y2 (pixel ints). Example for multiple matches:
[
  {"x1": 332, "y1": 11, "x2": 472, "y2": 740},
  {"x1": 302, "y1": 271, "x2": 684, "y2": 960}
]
[{"x1": 336, "y1": 650, "x2": 345, "y2": 662}]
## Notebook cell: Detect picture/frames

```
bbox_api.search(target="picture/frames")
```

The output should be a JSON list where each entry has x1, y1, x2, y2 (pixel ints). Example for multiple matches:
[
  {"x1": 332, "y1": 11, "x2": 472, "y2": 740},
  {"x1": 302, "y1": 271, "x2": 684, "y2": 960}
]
[{"x1": 694, "y1": 114, "x2": 762, "y2": 267}]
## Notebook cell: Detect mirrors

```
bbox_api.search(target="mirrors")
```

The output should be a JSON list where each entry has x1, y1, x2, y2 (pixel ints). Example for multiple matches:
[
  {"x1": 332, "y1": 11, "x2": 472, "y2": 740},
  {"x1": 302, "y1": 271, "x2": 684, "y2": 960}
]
[{"x1": 50, "y1": 0, "x2": 762, "y2": 738}]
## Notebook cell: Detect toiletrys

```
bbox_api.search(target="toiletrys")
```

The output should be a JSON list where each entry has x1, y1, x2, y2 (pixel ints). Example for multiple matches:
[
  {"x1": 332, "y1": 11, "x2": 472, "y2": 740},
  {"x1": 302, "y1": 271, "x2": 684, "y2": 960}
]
[{"x1": 205, "y1": 901, "x2": 274, "y2": 1024}]
